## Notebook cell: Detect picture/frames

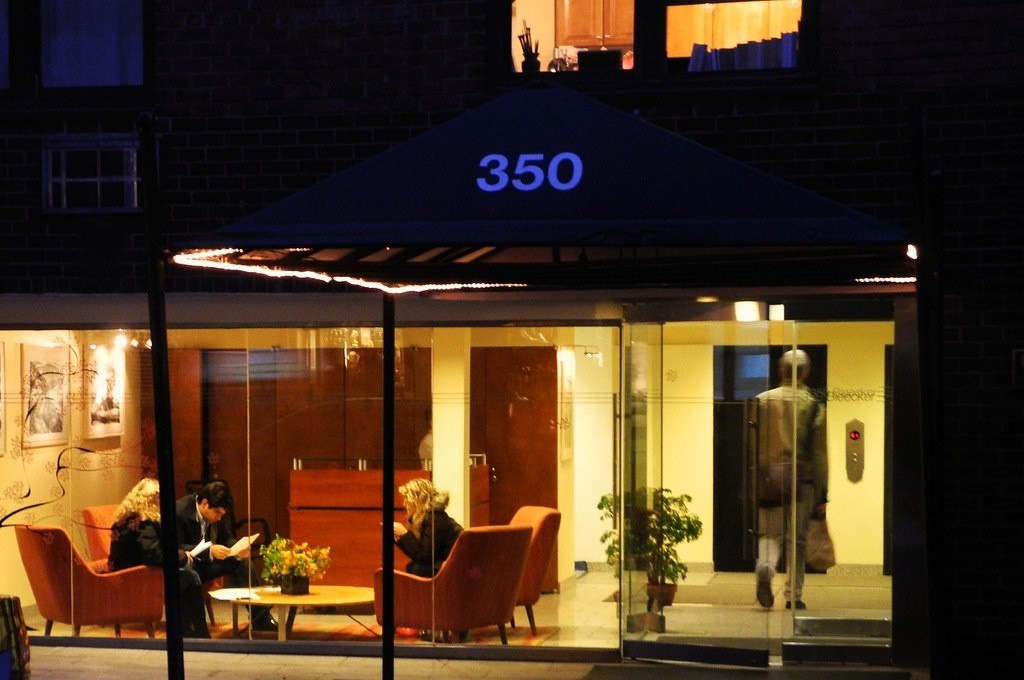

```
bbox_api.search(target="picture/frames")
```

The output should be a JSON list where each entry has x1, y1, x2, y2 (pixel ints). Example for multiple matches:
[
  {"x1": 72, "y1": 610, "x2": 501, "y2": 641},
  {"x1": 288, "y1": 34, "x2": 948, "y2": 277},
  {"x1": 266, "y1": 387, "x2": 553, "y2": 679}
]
[
  {"x1": 83, "y1": 342, "x2": 125, "y2": 440},
  {"x1": 20, "y1": 342, "x2": 71, "y2": 449}
]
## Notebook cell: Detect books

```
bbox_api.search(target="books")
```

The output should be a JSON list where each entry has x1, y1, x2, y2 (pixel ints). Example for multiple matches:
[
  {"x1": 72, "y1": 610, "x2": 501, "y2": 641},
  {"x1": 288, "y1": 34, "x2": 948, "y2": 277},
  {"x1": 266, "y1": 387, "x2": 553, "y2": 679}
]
[{"x1": 688, "y1": 32, "x2": 797, "y2": 72}]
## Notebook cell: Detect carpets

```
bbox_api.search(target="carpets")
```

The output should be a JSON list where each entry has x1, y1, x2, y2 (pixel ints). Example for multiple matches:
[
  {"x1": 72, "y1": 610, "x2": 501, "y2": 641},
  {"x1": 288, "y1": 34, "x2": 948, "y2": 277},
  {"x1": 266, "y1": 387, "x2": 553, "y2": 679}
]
[{"x1": 81, "y1": 621, "x2": 560, "y2": 647}]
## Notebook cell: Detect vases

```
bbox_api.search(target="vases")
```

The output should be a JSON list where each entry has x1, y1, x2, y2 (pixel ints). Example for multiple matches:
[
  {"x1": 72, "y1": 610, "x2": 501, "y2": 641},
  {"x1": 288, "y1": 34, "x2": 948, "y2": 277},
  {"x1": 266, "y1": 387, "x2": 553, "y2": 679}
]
[{"x1": 279, "y1": 573, "x2": 310, "y2": 595}]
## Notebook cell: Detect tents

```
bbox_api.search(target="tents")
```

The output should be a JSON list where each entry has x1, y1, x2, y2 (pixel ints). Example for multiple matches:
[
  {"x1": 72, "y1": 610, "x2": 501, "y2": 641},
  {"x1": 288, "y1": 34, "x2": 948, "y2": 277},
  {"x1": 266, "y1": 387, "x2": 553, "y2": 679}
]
[{"x1": 153, "y1": 80, "x2": 955, "y2": 680}]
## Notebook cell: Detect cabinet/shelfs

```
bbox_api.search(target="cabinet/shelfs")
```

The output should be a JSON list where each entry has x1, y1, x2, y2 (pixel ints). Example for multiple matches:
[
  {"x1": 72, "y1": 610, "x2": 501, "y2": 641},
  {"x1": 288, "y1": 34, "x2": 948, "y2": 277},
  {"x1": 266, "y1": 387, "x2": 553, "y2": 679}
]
[{"x1": 554, "y1": 0, "x2": 634, "y2": 48}]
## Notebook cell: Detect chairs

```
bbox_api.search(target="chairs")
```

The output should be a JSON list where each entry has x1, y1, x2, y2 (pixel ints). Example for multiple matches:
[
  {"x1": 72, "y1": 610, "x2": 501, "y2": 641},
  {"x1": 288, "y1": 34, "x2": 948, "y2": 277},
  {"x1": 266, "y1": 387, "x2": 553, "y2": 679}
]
[
  {"x1": 374, "y1": 505, "x2": 562, "y2": 646},
  {"x1": 15, "y1": 503, "x2": 225, "y2": 638}
]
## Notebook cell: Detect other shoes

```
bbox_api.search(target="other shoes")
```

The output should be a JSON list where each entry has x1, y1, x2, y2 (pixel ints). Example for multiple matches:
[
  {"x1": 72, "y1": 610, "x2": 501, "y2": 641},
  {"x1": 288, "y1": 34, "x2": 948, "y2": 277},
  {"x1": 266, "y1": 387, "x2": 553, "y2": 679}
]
[
  {"x1": 756, "y1": 566, "x2": 774, "y2": 607},
  {"x1": 785, "y1": 600, "x2": 806, "y2": 609}
]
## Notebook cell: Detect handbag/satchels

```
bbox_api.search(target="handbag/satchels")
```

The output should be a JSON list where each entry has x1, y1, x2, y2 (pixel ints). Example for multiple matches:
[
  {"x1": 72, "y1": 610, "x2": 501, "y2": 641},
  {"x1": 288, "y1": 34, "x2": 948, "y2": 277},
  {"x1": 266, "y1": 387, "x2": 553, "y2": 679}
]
[
  {"x1": 803, "y1": 510, "x2": 835, "y2": 572},
  {"x1": 741, "y1": 462, "x2": 803, "y2": 507}
]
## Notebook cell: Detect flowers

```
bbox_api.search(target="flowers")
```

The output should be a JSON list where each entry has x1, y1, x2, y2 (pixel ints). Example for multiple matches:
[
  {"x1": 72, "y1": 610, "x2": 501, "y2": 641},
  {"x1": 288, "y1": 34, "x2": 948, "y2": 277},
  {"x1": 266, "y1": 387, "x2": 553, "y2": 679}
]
[{"x1": 259, "y1": 533, "x2": 332, "y2": 587}]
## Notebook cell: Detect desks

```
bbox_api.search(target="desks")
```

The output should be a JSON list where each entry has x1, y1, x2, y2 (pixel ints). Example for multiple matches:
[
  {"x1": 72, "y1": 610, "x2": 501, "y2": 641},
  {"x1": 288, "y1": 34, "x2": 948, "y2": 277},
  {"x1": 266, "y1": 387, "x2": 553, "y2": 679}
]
[{"x1": 207, "y1": 585, "x2": 375, "y2": 642}]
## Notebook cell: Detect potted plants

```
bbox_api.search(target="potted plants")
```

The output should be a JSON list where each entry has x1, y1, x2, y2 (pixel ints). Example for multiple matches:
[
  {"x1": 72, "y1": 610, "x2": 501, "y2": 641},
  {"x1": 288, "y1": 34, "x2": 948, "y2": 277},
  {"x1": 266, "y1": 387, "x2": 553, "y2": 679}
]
[{"x1": 597, "y1": 487, "x2": 703, "y2": 606}]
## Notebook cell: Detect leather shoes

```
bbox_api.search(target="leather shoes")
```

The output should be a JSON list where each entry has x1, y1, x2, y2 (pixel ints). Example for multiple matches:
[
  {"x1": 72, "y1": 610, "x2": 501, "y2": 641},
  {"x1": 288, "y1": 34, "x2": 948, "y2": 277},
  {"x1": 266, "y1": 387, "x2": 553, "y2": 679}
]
[{"x1": 251, "y1": 614, "x2": 278, "y2": 631}]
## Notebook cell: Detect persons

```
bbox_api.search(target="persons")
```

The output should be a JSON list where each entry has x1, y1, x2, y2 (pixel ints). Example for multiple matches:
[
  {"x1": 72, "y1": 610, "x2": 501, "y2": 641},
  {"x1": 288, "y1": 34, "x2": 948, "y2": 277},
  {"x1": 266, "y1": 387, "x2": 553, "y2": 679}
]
[
  {"x1": 394, "y1": 479, "x2": 470, "y2": 638},
  {"x1": 752, "y1": 349, "x2": 828, "y2": 608},
  {"x1": 176, "y1": 483, "x2": 278, "y2": 637},
  {"x1": 418, "y1": 408, "x2": 433, "y2": 470},
  {"x1": 110, "y1": 478, "x2": 211, "y2": 638}
]
